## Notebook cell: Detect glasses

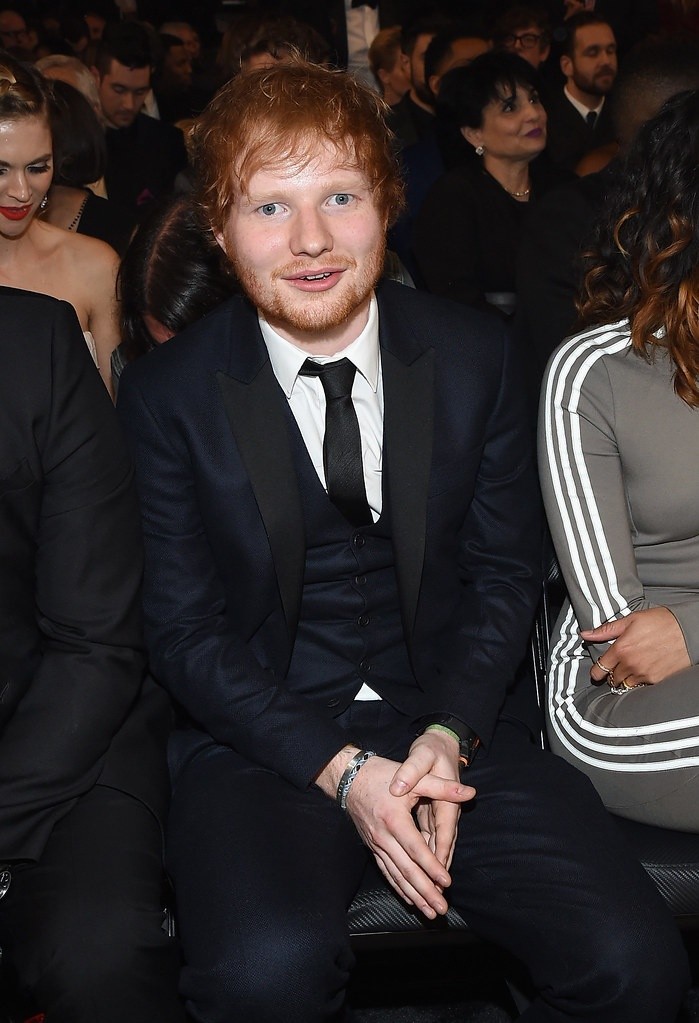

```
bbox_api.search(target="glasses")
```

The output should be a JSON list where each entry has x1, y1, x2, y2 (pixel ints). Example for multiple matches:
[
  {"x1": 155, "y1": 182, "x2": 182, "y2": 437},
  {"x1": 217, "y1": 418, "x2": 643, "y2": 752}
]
[{"x1": 503, "y1": 33, "x2": 543, "y2": 50}]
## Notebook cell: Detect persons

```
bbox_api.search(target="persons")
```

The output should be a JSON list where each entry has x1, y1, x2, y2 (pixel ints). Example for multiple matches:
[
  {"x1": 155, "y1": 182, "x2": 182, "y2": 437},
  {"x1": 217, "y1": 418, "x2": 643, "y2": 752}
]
[
  {"x1": 0, "y1": 0, "x2": 699, "y2": 1023},
  {"x1": 122, "y1": 58, "x2": 693, "y2": 1023}
]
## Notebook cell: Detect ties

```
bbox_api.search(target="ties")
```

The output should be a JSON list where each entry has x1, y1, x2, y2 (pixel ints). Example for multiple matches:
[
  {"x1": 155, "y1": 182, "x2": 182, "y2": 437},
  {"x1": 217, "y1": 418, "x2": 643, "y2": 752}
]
[
  {"x1": 585, "y1": 111, "x2": 597, "y2": 144},
  {"x1": 301, "y1": 357, "x2": 375, "y2": 527}
]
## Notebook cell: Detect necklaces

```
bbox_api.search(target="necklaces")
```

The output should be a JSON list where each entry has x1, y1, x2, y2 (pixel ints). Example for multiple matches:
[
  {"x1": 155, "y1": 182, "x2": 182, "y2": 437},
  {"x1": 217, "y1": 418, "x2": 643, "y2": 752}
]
[
  {"x1": 512, "y1": 190, "x2": 529, "y2": 196},
  {"x1": 67, "y1": 196, "x2": 87, "y2": 230}
]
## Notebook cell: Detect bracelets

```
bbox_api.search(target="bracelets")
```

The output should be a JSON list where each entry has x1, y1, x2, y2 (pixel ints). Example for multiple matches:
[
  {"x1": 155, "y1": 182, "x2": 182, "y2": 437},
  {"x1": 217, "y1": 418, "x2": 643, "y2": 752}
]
[
  {"x1": 337, "y1": 751, "x2": 373, "y2": 808},
  {"x1": 426, "y1": 725, "x2": 460, "y2": 742}
]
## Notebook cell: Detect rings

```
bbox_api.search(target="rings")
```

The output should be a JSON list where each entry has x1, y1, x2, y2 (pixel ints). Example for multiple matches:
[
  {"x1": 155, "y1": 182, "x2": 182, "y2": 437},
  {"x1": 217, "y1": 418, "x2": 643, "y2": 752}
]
[
  {"x1": 623, "y1": 680, "x2": 634, "y2": 690},
  {"x1": 596, "y1": 658, "x2": 613, "y2": 673}
]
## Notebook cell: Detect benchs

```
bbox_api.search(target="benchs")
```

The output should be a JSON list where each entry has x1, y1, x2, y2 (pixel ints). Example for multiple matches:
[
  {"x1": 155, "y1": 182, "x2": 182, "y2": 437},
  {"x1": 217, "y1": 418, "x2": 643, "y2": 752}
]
[{"x1": 163, "y1": 805, "x2": 699, "y2": 1023}]
